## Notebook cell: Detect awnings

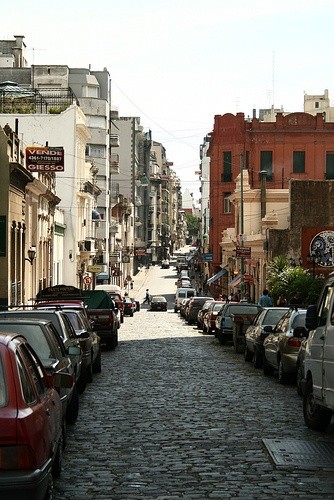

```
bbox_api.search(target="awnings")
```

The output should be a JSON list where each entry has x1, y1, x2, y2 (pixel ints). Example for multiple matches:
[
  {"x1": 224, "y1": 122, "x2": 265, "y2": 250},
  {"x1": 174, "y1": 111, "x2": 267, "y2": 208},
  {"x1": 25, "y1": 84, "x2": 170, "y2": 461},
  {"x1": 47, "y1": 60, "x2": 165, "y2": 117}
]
[
  {"x1": 228, "y1": 274, "x2": 245, "y2": 289},
  {"x1": 207, "y1": 269, "x2": 228, "y2": 291}
]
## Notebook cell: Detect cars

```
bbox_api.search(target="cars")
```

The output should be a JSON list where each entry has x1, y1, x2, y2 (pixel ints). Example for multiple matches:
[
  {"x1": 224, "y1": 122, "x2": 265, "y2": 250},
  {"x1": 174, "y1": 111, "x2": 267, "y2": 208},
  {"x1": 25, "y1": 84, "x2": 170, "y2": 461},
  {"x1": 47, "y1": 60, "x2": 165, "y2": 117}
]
[
  {"x1": 244, "y1": 306, "x2": 290, "y2": 369},
  {"x1": 0, "y1": 332, "x2": 74, "y2": 500},
  {"x1": 0, "y1": 283, "x2": 142, "y2": 396},
  {"x1": 293, "y1": 326, "x2": 310, "y2": 397},
  {"x1": 149, "y1": 296, "x2": 167, "y2": 312},
  {"x1": 174, "y1": 255, "x2": 262, "y2": 353},
  {"x1": 261, "y1": 306, "x2": 307, "y2": 385},
  {"x1": 0, "y1": 317, "x2": 82, "y2": 450}
]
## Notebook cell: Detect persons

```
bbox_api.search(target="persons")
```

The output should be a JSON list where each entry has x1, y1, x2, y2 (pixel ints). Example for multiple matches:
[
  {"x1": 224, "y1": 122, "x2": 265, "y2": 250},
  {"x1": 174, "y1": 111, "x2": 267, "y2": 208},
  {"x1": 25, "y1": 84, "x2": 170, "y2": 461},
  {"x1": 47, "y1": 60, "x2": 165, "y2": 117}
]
[
  {"x1": 166, "y1": 254, "x2": 170, "y2": 261},
  {"x1": 259, "y1": 290, "x2": 305, "y2": 307},
  {"x1": 143, "y1": 289, "x2": 150, "y2": 305},
  {"x1": 221, "y1": 290, "x2": 241, "y2": 302}
]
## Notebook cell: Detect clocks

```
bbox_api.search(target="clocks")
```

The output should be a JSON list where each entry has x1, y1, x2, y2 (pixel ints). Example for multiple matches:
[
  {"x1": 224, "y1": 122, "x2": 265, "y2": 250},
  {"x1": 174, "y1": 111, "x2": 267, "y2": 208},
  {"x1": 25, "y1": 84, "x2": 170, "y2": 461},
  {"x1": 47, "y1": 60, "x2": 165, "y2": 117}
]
[{"x1": 122, "y1": 255, "x2": 130, "y2": 263}]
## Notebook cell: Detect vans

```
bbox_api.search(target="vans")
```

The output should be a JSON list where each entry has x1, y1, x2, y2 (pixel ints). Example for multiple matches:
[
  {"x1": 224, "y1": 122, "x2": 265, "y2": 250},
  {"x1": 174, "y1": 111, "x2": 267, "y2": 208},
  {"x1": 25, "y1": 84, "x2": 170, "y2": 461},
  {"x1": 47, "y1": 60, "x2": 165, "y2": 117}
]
[{"x1": 297, "y1": 272, "x2": 333, "y2": 432}]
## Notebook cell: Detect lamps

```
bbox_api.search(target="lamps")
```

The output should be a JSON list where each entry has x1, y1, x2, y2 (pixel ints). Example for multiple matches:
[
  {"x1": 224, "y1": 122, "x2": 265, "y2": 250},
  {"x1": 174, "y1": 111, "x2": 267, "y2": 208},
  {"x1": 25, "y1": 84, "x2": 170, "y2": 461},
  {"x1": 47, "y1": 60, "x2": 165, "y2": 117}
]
[{"x1": 25, "y1": 250, "x2": 35, "y2": 265}]
